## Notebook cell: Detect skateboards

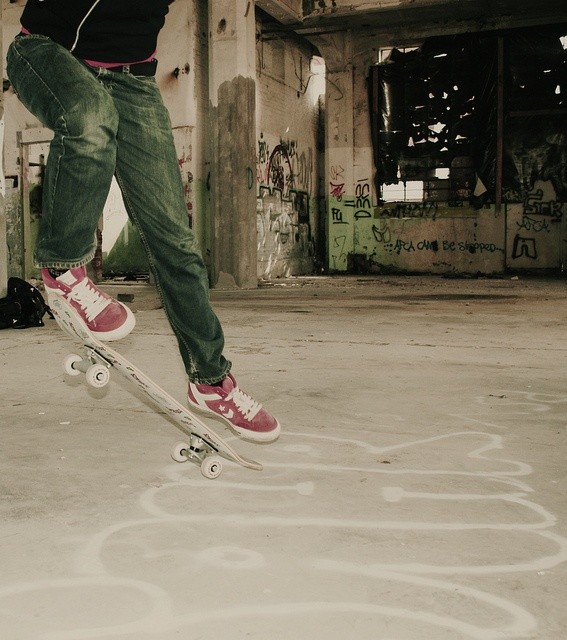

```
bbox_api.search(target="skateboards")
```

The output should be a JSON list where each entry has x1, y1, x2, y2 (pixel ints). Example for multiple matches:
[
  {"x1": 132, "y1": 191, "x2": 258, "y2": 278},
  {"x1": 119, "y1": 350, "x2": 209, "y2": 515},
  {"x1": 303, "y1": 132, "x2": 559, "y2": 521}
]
[{"x1": 47, "y1": 292, "x2": 263, "y2": 478}]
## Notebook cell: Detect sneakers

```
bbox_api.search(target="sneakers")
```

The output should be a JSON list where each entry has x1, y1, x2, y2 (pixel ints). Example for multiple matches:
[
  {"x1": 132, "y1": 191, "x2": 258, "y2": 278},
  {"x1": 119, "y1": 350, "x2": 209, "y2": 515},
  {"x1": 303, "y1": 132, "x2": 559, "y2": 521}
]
[
  {"x1": 187, "y1": 380, "x2": 282, "y2": 444},
  {"x1": 45, "y1": 265, "x2": 138, "y2": 344}
]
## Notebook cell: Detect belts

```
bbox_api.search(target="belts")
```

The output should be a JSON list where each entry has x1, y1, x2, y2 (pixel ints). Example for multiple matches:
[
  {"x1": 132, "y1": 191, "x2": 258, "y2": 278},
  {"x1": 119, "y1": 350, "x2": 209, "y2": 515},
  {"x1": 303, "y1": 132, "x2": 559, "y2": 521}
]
[{"x1": 102, "y1": 58, "x2": 158, "y2": 79}]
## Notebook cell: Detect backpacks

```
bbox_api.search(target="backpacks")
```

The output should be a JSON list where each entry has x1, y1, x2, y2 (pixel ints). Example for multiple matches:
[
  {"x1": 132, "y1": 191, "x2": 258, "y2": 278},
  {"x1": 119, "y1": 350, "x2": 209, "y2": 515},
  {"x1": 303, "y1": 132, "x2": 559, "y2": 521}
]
[{"x1": 7, "y1": 277, "x2": 55, "y2": 329}]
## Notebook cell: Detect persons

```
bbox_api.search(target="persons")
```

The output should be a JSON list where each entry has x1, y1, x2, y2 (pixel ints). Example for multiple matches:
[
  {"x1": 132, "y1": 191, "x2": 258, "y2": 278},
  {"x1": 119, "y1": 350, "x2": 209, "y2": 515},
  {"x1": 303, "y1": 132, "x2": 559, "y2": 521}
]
[{"x1": 6, "y1": 0, "x2": 281, "y2": 443}]
